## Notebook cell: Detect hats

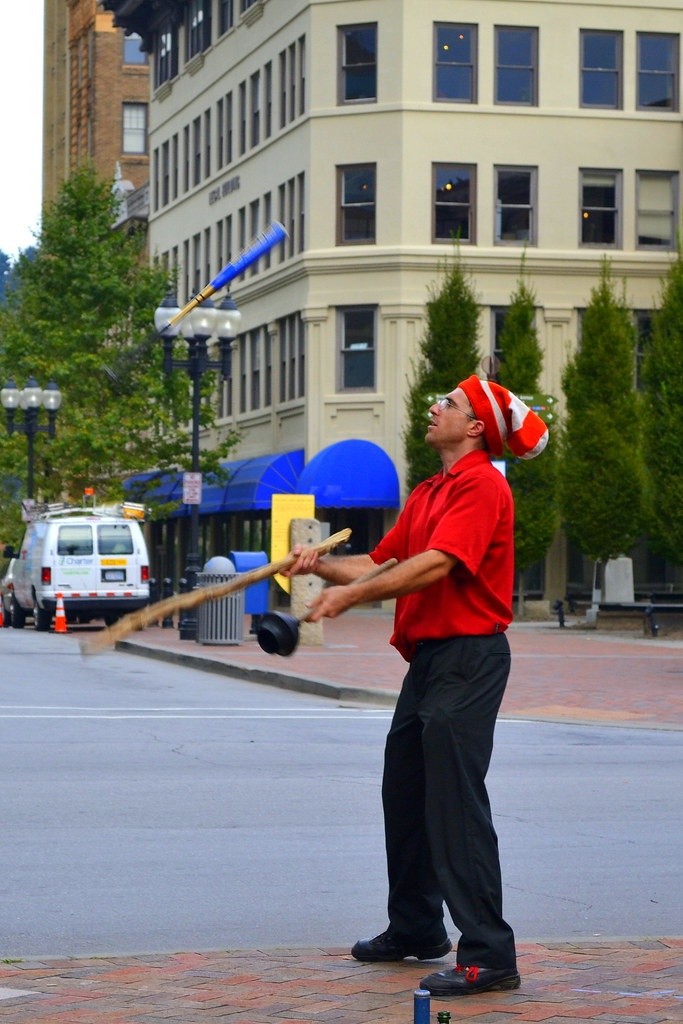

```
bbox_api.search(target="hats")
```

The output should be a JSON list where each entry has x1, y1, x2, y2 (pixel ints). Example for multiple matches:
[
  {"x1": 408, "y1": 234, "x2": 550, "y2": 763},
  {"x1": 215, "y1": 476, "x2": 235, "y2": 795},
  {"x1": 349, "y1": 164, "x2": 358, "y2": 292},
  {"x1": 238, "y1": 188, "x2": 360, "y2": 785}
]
[{"x1": 459, "y1": 375, "x2": 549, "y2": 461}]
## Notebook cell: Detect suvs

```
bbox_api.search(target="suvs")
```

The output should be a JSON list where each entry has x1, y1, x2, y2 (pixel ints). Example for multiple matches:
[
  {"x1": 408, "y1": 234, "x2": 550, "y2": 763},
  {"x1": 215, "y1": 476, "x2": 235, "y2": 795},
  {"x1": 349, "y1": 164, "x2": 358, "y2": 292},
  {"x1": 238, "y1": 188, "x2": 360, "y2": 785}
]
[{"x1": 3, "y1": 506, "x2": 152, "y2": 631}]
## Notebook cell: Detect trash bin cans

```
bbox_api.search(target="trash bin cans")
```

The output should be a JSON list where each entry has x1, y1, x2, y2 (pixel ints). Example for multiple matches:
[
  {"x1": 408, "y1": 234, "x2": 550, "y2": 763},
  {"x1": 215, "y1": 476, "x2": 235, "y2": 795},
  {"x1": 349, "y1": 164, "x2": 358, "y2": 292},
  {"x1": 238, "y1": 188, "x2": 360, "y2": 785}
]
[
  {"x1": 195, "y1": 572, "x2": 246, "y2": 644},
  {"x1": 228, "y1": 550, "x2": 270, "y2": 615}
]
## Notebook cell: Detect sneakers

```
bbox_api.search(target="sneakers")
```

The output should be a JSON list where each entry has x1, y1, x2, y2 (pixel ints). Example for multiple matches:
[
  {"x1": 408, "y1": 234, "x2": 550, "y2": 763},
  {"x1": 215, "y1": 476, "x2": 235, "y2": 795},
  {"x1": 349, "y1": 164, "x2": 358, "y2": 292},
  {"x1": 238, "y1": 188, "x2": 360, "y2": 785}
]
[
  {"x1": 351, "y1": 926, "x2": 452, "y2": 960},
  {"x1": 420, "y1": 965, "x2": 521, "y2": 996}
]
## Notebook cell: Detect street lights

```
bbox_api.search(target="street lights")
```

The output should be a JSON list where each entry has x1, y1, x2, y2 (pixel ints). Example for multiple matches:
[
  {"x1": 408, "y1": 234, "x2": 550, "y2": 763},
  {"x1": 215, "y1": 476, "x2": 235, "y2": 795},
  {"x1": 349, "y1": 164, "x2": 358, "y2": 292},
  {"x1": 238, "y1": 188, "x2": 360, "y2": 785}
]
[
  {"x1": 0, "y1": 387, "x2": 62, "y2": 511},
  {"x1": 153, "y1": 292, "x2": 241, "y2": 641},
  {"x1": 48, "y1": 592, "x2": 73, "y2": 634}
]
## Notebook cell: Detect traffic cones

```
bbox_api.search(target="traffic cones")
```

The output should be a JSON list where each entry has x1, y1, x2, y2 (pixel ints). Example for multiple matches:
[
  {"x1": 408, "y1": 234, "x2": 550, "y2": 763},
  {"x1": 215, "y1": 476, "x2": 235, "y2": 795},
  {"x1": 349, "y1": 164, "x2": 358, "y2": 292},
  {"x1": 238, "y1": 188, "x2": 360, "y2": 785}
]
[{"x1": 0, "y1": 593, "x2": 6, "y2": 628}]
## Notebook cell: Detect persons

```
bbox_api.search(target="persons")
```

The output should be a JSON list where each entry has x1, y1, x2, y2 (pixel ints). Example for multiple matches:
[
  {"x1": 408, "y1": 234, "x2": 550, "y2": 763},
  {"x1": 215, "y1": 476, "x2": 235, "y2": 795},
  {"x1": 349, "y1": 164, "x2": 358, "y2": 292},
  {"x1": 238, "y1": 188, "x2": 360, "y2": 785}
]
[{"x1": 283, "y1": 374, "x2": 551, "y2": 995}]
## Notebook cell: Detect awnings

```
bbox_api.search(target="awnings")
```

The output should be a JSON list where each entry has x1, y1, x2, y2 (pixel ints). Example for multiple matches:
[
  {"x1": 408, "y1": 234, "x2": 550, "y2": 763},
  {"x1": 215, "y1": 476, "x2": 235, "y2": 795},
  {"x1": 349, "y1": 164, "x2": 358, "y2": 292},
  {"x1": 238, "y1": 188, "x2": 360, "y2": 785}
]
[
  {"x1": 123, "y1": 449, "x2": 305, "y2": 517},
  {"x1": 295, "y1": 439, "x2": 401, "y2": 510}
]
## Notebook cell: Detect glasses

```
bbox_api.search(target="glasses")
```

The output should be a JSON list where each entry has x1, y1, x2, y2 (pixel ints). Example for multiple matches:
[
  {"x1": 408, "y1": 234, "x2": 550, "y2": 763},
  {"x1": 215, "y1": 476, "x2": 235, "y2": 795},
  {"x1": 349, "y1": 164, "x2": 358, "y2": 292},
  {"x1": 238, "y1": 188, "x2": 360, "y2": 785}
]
[{"x1": 438, "y1": 398, "x2": 477, "y2": 421}]
